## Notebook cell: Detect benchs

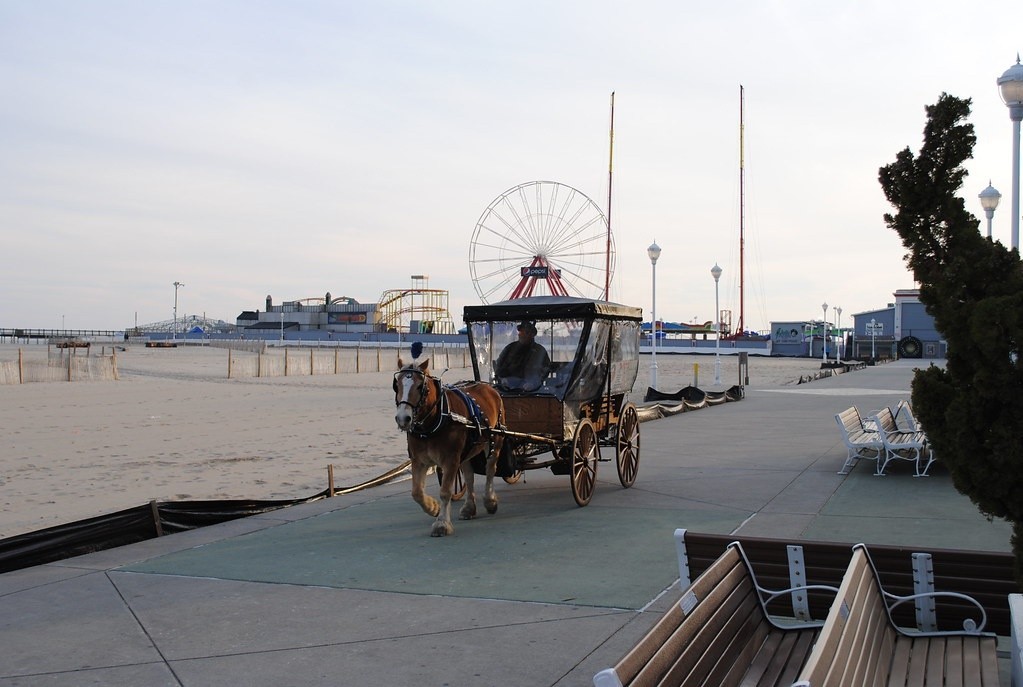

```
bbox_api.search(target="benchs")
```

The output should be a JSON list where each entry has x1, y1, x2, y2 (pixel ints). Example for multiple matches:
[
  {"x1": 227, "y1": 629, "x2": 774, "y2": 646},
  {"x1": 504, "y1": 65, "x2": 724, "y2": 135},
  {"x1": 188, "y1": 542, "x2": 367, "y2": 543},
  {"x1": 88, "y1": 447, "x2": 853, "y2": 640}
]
[
  {"x1": 675, "y1": 526, "x2": 1022, "y2": 636},
  {"x1": 836, "y1": 404, "x2": 888, "y2": 477},
  {"x1": 900, "y1": 399, "x2": 924, "y2": 432},
  {"x1": 858, "y1": 399, "x2": 903, "y2": 434},
  {"x1": 792, "y1": 542, "x2": 1001, "y2": 687},
  {"x1": 592, "y1": 542, "x2": 839, "y2": 687},
  {"x1": 873, "y1": 406, "x2": 930, "y2": 478},
  {"x1": 535, "y1": 362, "x2": 608, "y2": 399}
]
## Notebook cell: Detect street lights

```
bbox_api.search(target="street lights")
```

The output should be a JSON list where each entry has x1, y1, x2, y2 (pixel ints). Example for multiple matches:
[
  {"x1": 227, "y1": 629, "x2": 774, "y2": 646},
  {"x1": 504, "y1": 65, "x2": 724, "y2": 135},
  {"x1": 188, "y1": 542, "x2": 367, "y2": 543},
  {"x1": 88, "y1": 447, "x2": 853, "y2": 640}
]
[
  {"x1": 997, "y1": 52, "x2": 1023, "y2": 255},
  {"x1": 978, "y1": 180, "x2": 1002, "y2": 236},
  {"x1": 837, "y1": 306, "x2": 843, "y2": 364},
  {"x1": 173, "y1": 281, "x2": 184, "y2": 340},
  {"x1": 871, "y1": 318, "x2": 876, "y2": 358},
  {"x1": 647, "y1": 239, "x2": 662, "y2": 390},
  {"x1": 711, "y1": 262, "x2": 723, "y2": 384},
  {"x1": 822, "y1": 302, "x2": 828, "y2": 363}
]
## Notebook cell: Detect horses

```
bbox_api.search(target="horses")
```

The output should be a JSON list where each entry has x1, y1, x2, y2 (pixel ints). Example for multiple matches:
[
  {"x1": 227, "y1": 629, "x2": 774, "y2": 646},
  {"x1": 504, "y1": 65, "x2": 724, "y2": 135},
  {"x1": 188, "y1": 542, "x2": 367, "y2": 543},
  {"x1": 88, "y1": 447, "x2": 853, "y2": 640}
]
[{"x1": 393, "y1": 358, "x2": 506, "y2": 537}]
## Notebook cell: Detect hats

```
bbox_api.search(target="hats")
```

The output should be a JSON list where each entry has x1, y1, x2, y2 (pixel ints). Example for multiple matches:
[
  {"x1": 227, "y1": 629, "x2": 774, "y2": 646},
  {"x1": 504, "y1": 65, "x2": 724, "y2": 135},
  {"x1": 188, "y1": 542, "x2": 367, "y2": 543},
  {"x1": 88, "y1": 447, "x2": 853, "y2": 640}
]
[{"x1": 517, "y1": 321, "x2": 538, "y2": 336}]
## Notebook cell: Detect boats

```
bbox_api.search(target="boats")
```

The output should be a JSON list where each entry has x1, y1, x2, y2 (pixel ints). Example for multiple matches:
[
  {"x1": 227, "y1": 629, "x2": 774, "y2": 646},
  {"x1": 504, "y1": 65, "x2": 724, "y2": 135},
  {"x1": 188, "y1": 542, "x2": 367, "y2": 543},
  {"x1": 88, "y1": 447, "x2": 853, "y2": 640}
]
[
  {"x1": 144, "y1": 341, "x2": 177, "y2": 348},
  {"x1": 57, "y1": 340, "x2": 91, "y2": 348}
]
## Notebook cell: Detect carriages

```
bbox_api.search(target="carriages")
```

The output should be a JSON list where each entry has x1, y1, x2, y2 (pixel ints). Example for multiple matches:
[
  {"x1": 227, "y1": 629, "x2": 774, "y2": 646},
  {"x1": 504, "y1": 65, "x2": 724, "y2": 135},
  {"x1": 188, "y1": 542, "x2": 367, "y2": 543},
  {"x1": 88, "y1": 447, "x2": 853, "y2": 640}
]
[{"x1": 392, "y1": 295, "x2": 642, "y2": 537}]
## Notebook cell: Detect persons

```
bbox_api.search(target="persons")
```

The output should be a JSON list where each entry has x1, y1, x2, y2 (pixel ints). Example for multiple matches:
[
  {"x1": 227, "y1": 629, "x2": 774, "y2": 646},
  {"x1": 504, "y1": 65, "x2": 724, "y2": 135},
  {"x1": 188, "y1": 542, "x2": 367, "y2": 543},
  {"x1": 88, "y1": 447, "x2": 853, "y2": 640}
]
[
  {"x1": 825, "y1": 345, "x2": 831, "y2": 358},
  {"x1": 494, "y1": 322, "x2": 553, "y2": 394}
]
